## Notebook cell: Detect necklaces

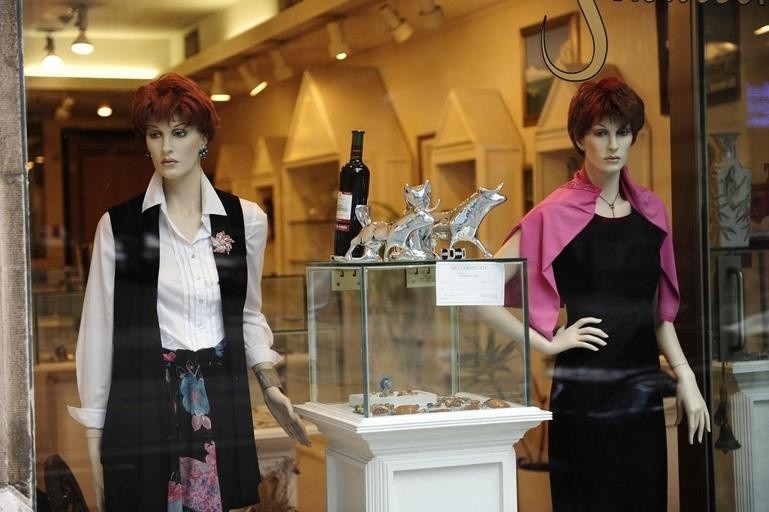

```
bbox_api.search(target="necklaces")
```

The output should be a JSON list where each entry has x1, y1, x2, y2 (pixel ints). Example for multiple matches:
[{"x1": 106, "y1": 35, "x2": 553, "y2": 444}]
[{"x1": 600, "y1": 192, "x2": 619, "y2": 218}]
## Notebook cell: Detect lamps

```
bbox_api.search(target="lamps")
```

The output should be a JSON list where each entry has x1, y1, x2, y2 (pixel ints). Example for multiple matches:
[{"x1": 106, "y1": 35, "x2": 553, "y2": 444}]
[
  {"x1": 59, "y1": 1, "x2": 93, "y2": 55},
  {"x1": 36, "y1": 28, "x2": 63, "y2": 68},
  {"x1": 53, "y1": 96, "x2": 112, "y2": 122},
  {"x1": 209, "y1": 0, "x2": 445, "y2": 103}
]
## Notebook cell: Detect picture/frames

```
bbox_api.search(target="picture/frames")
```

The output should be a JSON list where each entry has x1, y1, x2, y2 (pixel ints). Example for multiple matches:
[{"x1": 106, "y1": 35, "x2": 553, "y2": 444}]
[{"x1": 519, "y1": 11, "x2": 580, "y2": 127}]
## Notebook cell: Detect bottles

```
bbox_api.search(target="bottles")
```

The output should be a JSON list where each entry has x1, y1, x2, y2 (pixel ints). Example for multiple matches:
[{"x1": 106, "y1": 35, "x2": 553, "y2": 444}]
[{"x1": 332, "y1": 129, "x2": 372, "y2": 258}]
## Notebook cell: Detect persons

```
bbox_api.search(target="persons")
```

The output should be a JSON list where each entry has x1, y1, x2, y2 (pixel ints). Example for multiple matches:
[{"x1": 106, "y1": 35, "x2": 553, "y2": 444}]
[
  {"x1": 464, "y1": 74, "x2": 711, "y2": 511},
  {"x1": 67, "y1": 72, "x2": 313, "y2": 512}
]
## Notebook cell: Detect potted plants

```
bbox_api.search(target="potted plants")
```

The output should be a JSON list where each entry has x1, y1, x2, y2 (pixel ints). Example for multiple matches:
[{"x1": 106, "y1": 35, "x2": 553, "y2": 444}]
[{"x1": 450, "y1": 328, "x2": 554, "y2": 512}]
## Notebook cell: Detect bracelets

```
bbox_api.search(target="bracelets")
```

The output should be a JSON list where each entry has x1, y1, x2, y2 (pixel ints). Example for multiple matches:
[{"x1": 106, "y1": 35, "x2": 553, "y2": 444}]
[{"x1": 254, "y1": 367, "x2": 287, "y2": 395}]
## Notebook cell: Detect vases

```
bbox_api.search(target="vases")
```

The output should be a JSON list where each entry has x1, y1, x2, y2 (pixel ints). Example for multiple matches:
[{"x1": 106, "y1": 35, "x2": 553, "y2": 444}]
[{"x1": 707, "y1": 130, "x2": 751, "y2": 249}]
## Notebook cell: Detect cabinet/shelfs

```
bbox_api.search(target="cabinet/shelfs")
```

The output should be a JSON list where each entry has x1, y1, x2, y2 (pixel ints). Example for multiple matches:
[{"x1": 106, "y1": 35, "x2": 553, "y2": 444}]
[{"x1": 287, "y1": 216, "x2": 336, "y2": 267}]
